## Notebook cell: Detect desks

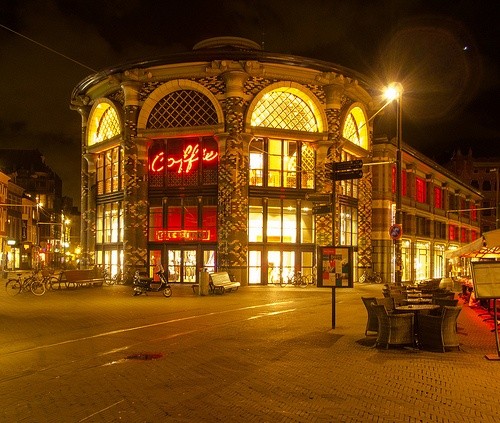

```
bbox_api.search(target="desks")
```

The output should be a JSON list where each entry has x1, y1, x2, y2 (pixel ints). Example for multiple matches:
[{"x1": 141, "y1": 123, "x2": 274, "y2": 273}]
[
  {"x1": 395, "y1": 304, "x2": 441, "y2": 350},
  {"x1": 407, "y1": 293, "x2": 433, "y2": 298},
  {"x1": 402, "y1": 298, "x2": 433, "y2": 304}
]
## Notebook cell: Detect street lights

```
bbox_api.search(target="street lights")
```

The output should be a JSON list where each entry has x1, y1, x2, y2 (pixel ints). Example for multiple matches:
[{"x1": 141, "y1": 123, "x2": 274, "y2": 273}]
[{"x1": 379, "y1": 81, "x2": 404, "y2": 283}]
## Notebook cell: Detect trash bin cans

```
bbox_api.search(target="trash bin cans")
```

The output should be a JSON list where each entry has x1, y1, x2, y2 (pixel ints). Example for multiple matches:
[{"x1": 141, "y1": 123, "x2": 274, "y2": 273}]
[
  {"x1": 94, "y1": 267, "x2": 103, "y2": 286},
  {"x1": 4, "y1": 270, "x2": 9, "y2": 279},
  {"x1": 199, "y1": 268, "x2": 209, "y2": 296}
]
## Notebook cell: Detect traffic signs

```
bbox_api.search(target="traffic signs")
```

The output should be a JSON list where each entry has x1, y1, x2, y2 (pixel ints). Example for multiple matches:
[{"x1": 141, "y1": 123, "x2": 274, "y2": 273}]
[
  {"x1": 324, "y1": 160, "x2": 363, "y2": 171},
  {"x1": 312, "y1": 205, "x2": 331, "y2": 214},
  {"x1": 325, "y1": 170, "x2": 364, "y2": 181},
  {"x1": 305, "y1": 193, "x2": 331, "y2": 202},
  {"x1": 338, "y1": 195, "x2": 359, "y2": 208}
]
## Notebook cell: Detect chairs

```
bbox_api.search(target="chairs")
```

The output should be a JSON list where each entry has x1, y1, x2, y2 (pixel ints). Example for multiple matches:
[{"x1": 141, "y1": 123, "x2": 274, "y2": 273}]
[{"x1": 360, "y1": 278, "x2": 462, "y2": 353}]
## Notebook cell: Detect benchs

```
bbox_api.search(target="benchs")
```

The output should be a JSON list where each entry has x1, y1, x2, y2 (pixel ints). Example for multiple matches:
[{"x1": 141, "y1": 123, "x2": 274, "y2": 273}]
[
  {"x1": 49, "y1": 269, "x2": 106, "y2": 289},
  {"x1": 190, "y1": 271, "x2": 240, "y2": 295}
]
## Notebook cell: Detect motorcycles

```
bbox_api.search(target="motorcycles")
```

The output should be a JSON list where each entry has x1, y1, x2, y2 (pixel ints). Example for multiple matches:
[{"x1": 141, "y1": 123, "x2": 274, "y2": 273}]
[{"x1": 133, "y1": 265, "x2": 172, "y2": 298}]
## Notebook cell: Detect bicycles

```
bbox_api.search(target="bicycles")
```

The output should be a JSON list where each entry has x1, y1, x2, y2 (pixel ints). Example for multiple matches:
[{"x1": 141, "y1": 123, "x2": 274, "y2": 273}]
[
  {"x1": 5, "y1": 268, "x2": 61, "y2": 296},
  {"x1": 358, "y1": 270, "x2": 383, "y2": 284},
  {"x1": 271, "y1": 271, "x2": 315, "y2": 288}
]
